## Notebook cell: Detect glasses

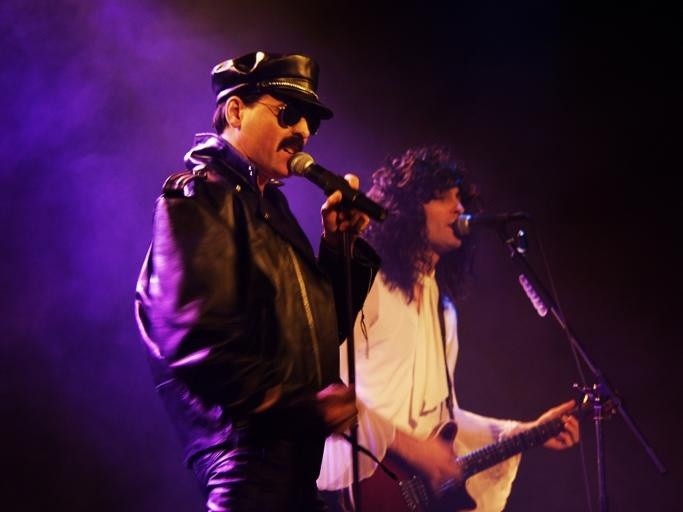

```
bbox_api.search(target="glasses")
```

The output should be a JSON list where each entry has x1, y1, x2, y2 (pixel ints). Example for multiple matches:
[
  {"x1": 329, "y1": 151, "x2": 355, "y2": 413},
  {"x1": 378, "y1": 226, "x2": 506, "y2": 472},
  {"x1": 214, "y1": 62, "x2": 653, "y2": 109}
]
[{"x1": 259, "y1": 102, "x2": 321, "y2": 134}]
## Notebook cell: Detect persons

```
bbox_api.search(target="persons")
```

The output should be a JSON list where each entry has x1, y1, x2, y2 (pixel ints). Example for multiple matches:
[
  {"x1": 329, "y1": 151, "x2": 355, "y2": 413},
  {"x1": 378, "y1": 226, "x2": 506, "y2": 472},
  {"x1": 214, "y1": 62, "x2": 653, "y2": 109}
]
[
  {"x1": 133, "y1": 49, "x2": 369, "y2": 497},
  {"x1": 316, "y1": 145, "x2": 581, "y2": 512}
]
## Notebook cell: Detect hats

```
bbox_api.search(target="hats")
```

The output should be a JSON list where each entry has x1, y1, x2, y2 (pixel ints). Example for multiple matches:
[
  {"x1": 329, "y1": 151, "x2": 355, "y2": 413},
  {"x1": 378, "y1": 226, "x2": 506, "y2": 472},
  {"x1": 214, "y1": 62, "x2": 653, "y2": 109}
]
[{"x1": 210, "y1": 50, "x2": 333, "y2": 120}]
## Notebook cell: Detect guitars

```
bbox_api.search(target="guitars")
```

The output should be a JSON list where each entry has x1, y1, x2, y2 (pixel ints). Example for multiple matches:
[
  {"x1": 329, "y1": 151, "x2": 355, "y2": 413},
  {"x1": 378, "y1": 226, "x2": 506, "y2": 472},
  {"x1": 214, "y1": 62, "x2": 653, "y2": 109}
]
[{"x1": 340, "y1": 380, "x2": 620, "y2": 512}]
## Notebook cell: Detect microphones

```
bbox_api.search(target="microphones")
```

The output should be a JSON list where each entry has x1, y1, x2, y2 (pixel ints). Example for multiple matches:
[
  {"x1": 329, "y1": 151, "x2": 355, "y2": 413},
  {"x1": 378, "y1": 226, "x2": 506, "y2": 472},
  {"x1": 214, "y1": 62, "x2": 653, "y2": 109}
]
[
  {"x1": 455, "y1": 210, "x2": 522, "y2": 236},
  {"x1": 287, "y1": 150, "x2": 388, "y2": 222}
]
